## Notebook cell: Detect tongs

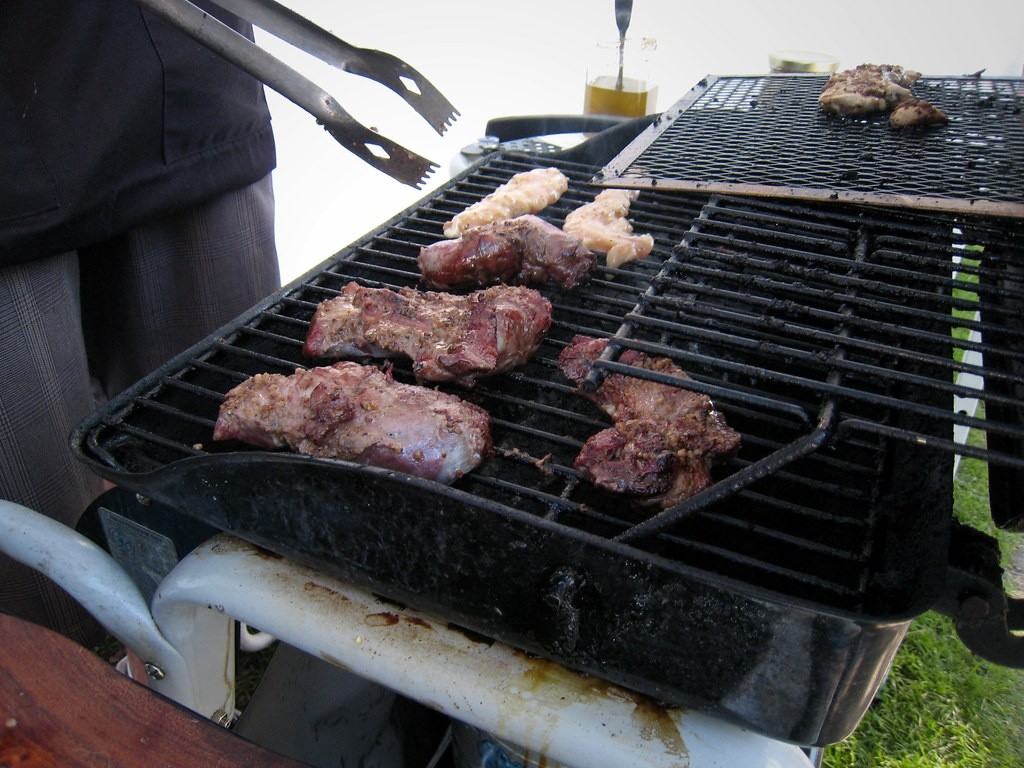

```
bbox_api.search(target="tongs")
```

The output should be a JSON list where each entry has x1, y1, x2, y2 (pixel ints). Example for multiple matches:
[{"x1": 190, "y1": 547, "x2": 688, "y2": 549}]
[{"x1": 142, "y1": 0, "x2": 461, "y2": 191}]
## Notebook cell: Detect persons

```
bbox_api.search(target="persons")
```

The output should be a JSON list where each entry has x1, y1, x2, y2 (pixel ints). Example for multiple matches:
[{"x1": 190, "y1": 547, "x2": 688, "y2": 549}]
[{"x1": 0, "y1": 0, "x2": 280, "y2": 658}]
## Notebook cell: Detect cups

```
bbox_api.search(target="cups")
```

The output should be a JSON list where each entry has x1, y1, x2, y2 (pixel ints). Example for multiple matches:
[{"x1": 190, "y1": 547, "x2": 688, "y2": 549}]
[
  {"x1": 582, "y1": 47, "x2": 660, "y2": 118},
  {"x1": 768, "y1": 49, "x2": 840, "y2": 73}
]
